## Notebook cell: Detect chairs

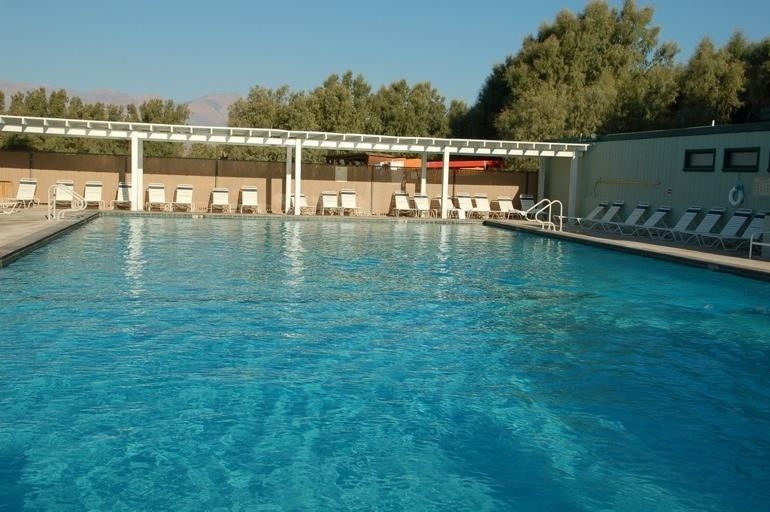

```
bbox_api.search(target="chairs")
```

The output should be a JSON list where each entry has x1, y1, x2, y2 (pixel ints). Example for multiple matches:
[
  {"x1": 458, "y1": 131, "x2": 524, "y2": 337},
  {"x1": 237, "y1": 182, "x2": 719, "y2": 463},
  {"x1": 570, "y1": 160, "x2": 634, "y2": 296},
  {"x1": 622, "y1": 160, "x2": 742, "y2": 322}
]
[
  {"x1": 289, "y1": 187, "x2": 357, "y2": 217},
  {"x1": 555, "y1": 201, "x2": 770, "y2": 252},
  {"x1": 392, "y1": 191, "x2": 548, "y2": 219},
  {"x1": 1, "y1": 177, "x2": 133, "y2": 212},
  {"x1": 147, "y1": 181, "x2": 260, "y2": 213}
]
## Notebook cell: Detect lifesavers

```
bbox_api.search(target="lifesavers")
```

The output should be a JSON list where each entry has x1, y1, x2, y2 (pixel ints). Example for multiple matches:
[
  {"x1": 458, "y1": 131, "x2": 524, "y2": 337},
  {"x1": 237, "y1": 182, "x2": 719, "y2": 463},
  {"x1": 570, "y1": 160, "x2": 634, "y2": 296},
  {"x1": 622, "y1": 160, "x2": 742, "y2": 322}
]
[{"x1": 728, "y1": 185, "x2": 744, "y2": 207}]
